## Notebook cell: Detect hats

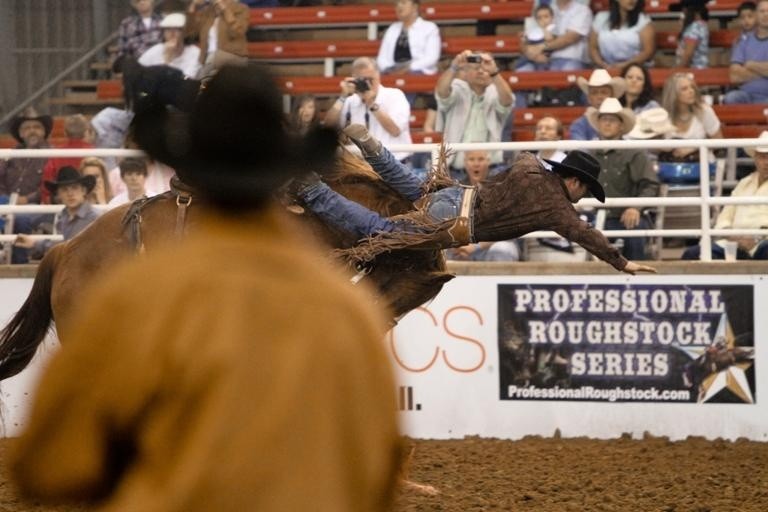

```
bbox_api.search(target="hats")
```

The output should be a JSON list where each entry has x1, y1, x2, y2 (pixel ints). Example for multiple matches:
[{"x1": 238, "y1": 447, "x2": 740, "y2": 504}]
[
  {"x1": 9, "y1": 106, "x2": 55, "y2": 144},
  {"x1": 575, "y1": 68, "x2": 627, "y2": 99},
  {"x1": 588, "y1": 96, "x2": 637, "y2": 135},
  {"x1": 629, "y1": 107, "x2": 679, "y2": 140},
  {"x1": 43, "y1": 165, "x2": 98, "y2": 197},
  {"x1": 157, "y1": 11, "x2": 189, "y2": 30},
  {"x1": 131, "y1": 58, "x2": 328, "y2": 221},
  {"x1": 541, "y1": 148, "x2": 606, "y2": 207},
  {"x1": 742, "y1": 129, "x2": 768, "y2": 159}
]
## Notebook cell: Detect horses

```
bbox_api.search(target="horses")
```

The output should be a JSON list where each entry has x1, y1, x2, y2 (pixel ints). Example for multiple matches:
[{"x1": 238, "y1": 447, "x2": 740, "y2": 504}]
[{"x1": 0, "y1": 142, "x2": 456, "y2": 384}]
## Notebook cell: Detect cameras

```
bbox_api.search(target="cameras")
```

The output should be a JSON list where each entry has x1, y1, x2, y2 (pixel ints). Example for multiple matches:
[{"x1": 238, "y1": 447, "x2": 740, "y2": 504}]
[
  {"x1": 466, "y1": 55, "x2": 481, "y2": 64},
  {"x1": 350, "y1": 77, "x2": 374, "y2": 93}
]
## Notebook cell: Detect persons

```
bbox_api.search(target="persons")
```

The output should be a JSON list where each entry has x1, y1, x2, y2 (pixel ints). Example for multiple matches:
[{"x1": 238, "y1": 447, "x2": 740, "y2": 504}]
[
  {"x1": 295, "y1": 2, "x2": 768, "y2": 273},
  {"x1": 14, "y1": 60, "x2": 404, "y2": 512},
  {"x1": 0, "y1": 0, "x2": 250, "y2": 264}
]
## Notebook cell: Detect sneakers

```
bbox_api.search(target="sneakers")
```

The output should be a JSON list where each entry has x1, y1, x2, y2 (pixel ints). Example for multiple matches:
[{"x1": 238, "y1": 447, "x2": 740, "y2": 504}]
[{"x1": 341, "y1": 122, "x2": 379, "y2": 154}]
